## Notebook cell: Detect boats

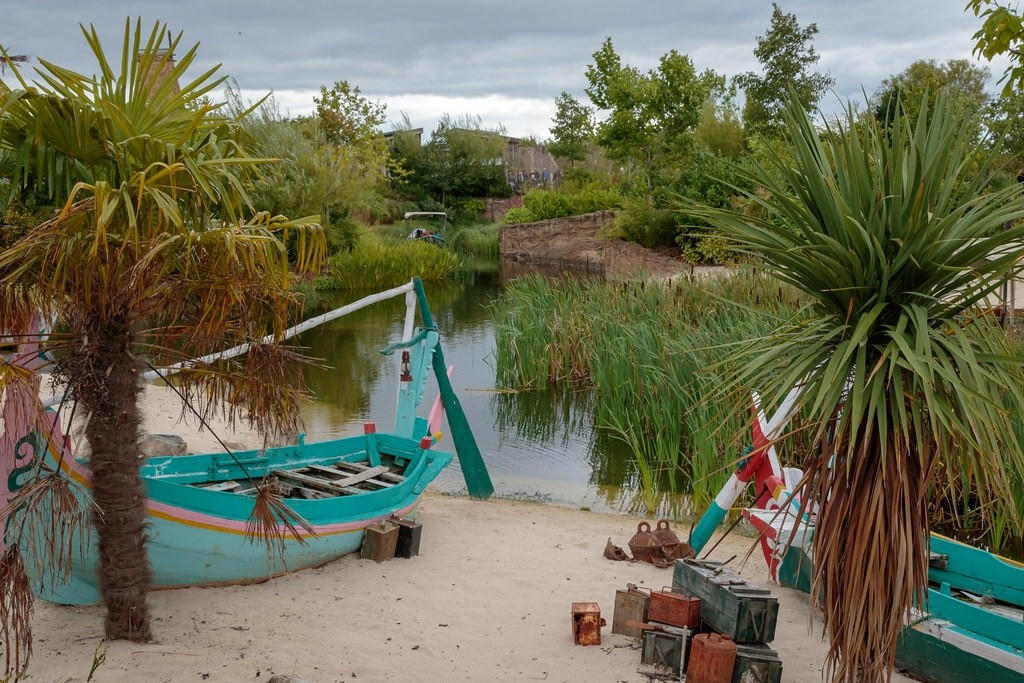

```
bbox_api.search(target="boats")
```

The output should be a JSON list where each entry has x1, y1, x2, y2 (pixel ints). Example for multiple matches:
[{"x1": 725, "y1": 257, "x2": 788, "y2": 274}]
[
  {"x1": 1, "y1": 274, "x2": 496, "y2": 608},
  {"x1": 684, "y1": 354, "x2": 1024, "y2": 682},
  {"x1": 405, "y1": 211, "x2": 448, "y2": 249}
]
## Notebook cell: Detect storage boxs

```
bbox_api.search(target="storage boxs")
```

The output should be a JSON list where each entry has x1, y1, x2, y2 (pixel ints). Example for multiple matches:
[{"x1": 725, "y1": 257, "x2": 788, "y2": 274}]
[
  {"x1": 387, "y1": 513, "x2": 421, "y2": 560},
  {"x1": 611, "y1": 558, "x2": 783, "y2": 683},
  {"x1": 572, "y1": 602, "x2": 607, "y2": 646},
  {"x1": 361, "y1": 518, "x2": 400, "y2": 563}
]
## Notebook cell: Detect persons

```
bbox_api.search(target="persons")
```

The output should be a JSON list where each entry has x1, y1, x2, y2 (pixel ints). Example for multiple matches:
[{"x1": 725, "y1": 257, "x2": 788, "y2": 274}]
[
  {"x1": 508, "y1": 167, "x2": 563, "y2": 190},
  {"x1": 415, "y1": 229, "x2": 441, "y2": 244}
]
